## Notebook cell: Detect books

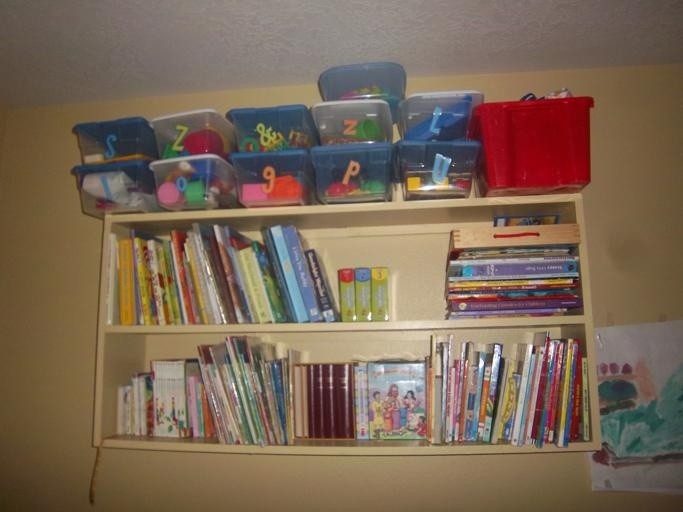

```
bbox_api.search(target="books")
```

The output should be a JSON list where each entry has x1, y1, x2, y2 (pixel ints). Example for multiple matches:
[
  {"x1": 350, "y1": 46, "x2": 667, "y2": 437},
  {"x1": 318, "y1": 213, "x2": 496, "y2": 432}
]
[
  {"x1": 106, "y1": 221, "x2": 392, "y2": 324},
  {"x1": 443, "y1": 214, "x2": 583, "y2": 319}
]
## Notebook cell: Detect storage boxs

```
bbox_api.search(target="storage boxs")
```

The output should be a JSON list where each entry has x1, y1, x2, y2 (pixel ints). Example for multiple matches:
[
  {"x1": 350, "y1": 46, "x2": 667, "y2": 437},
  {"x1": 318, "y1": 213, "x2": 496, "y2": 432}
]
[
  {"x1": 310, "y1": 143, "x2": 396, "y2": 204},
  {"x1": 229, "y1": 144, "x2": 311, "y2": 206},
  {"x1": 149, "y1": 152, "x2": 237, "y2": 210},
  {"x1": 398, "y1": 139, "x2": 481, "y2": 201},
  {"x1": 395, "y1": 91, "x2": 484, "y2": 143},
  {"x1": 151, "y1": 109, "x2": 237, "y2": 166},
  {"x1": 73, "y1": 116, "x2": 159, "y2": 168},
  {"x1": 468, "y1": 97, "x2": 594, "y2": 196},
  {"x1": 311, "y1": 98, "x2": 394, "y2": 145},
  {"x1": 226, "y1": 103, "x2": 318, "y2": 151},
  {"x1": 319, "y1": 62, "x2": 406, "y2": 123},
  {"x1": 71, "y1": 162, "x2": 157, "y2": 218}
]
